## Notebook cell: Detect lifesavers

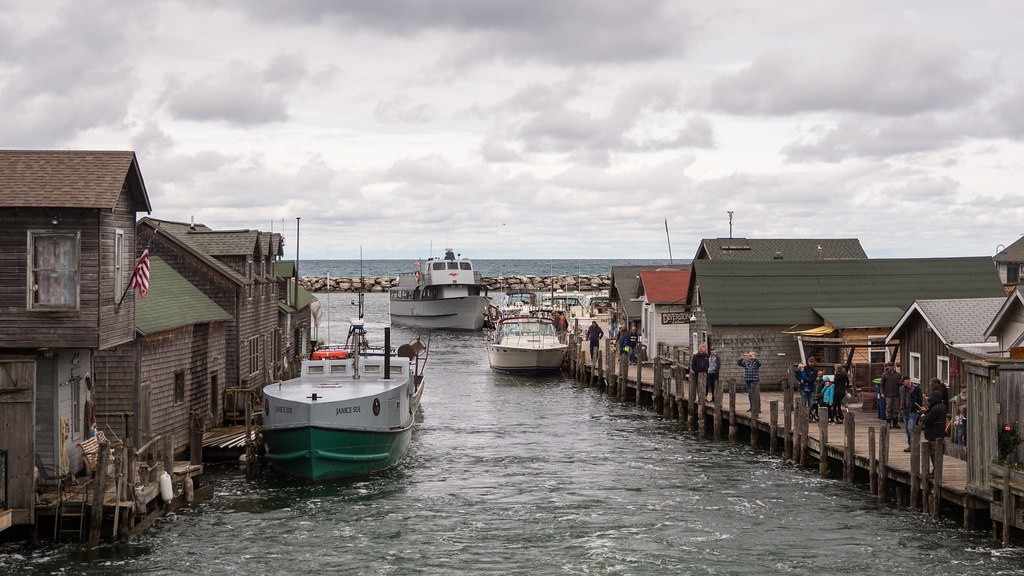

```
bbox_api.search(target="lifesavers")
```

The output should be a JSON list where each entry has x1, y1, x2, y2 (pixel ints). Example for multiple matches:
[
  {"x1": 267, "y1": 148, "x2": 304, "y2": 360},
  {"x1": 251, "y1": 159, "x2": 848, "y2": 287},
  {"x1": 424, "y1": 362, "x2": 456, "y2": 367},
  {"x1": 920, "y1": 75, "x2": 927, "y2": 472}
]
[{"x1": 415, "y1": 272, "x2": 419, "y2": 282}]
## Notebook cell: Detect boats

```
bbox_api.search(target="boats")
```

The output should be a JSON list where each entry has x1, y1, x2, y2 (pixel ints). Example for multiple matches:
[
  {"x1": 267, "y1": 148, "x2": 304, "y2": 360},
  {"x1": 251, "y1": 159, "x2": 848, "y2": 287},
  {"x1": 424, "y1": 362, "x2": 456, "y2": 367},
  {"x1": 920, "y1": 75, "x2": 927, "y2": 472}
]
[
  {"x1": 483, "y1": 257, "x2": 610, "y2": 375},
  {"x1": 259, "y1": 245, "x2": 431, "y2": 481},
  {"x1": 389, "y1": 240, "x2": 494, "y2": 332}
]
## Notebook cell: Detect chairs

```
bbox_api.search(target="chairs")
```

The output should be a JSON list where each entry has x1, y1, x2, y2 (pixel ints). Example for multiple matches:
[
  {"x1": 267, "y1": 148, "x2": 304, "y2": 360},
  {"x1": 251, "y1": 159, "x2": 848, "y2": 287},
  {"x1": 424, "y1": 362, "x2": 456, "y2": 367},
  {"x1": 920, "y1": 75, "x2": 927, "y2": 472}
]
[{"x1": 35, "y1": 453, "x2": 74, "y2": 501}]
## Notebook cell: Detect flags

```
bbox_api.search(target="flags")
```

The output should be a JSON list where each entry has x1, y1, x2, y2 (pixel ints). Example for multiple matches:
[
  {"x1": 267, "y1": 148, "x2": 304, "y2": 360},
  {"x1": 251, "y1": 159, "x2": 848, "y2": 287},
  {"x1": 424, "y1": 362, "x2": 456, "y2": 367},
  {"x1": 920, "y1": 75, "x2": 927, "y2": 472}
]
[
  {"x1": 133, "y1": 232, "x2": 156, "y2": 298},
  {"x1": 414, "y1": 260, "x2": 420, "y2": 266}
]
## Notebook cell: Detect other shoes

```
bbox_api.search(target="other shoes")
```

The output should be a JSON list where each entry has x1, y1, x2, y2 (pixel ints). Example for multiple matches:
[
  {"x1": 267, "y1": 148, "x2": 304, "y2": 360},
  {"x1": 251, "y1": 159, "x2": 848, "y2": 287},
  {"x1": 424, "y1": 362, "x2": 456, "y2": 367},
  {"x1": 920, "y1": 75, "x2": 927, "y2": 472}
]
[
  {"x1": 904, "y1": 446, "x2": 911, "y2": 452},
  {"x1": 710, "y1": 399, "x2": 714, "y2": 403}
]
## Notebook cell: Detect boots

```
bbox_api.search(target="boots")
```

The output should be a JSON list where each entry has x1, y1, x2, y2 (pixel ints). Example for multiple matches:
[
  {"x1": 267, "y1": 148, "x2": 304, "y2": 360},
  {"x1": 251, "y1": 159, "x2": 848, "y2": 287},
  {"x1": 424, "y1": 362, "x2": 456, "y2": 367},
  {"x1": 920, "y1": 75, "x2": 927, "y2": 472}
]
[
  {"x1": 887, "y1": 419, "x2": 891, "y2": 429},
  {"x1": 893, "y1": 417, "x2": 901, "y2": 429}
]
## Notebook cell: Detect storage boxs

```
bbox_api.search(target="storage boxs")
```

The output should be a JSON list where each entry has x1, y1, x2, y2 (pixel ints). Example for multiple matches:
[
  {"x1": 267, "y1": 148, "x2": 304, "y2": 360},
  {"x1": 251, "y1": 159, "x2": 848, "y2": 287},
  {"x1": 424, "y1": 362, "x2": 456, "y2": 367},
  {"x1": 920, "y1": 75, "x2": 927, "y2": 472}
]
[{"x1": 313, "y1": 350, "x2": 350, "y2": 361}]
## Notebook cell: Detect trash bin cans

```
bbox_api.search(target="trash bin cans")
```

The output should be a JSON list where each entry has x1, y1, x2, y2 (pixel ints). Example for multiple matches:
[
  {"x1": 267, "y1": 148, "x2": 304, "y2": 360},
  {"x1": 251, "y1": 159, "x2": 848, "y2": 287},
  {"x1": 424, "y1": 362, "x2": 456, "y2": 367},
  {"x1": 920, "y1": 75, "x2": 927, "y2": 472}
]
[{"x1": 872, "y1": 378, "x2": 887, "y2": 420}]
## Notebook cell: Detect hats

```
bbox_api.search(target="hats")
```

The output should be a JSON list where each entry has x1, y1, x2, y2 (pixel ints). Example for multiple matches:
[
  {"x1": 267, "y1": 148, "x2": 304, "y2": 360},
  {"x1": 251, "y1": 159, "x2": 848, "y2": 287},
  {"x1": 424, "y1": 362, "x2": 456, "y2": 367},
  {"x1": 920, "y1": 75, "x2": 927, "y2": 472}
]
[
  {"x1": 898, "y1": 375, "x2": 910, "y2": 382},
  {"x1": 710, "y1": 346, "x2": 716, "y2": 350},
  {"x1": 929, "y1": 377, "x2": 940, "y2": 385}
]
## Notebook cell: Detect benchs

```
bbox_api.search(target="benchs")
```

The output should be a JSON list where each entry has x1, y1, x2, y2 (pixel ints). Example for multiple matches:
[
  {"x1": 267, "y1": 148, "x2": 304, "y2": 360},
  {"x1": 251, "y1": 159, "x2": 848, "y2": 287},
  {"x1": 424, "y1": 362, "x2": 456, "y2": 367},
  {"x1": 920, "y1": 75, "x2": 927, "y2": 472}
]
[{"x1": 77, "y1": 438, "x2": 115, "y2": 477}]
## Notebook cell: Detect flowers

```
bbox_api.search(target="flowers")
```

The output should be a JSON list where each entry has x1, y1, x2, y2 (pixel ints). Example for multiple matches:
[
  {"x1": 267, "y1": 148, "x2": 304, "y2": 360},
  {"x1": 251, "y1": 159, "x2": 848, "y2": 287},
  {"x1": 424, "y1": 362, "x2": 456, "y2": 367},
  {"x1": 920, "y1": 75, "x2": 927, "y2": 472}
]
[{"x1": 999, "y1": 423, "x2": 1021, "y2": 461}]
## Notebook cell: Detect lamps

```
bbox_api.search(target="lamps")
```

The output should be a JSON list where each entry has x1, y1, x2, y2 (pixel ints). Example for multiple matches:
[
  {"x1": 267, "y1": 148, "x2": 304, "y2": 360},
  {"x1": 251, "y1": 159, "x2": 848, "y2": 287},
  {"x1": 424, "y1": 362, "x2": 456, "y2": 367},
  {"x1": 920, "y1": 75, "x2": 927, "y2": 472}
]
[{"x1": 690, "y1": 310, "x2": 701, "y2": 321}]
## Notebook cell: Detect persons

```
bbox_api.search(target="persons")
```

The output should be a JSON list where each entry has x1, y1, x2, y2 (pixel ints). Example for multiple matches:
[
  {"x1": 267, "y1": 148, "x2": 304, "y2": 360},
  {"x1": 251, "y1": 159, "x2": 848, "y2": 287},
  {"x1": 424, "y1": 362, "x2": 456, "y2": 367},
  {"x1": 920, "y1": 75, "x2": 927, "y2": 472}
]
[
  {"x1": 586, "y1": 321, "x2": 604, "y2": 362},
  {"x1": 899, "y1": 376, "x2": 923, "y2": 452},
  {"x1": 956, "y1": 383, "x2": 967, "y2": 445},
  {"x1": 880, "y1": 361, "x2": 901, "y2": 429},
  {"x1": 553, "y1": 311, "x2": 568, "y2": 331},
  {"x1": 920, "y1": 378, "x2": 949, "y2": 476},
  {"x1": 833, "y1": 364, "x2": 849, "y2": 423},
  {"x1": 795, "y1": 355, "x2": 825, "y2": 409},
  {"x1": 610, "y1": 308, "x2": 617, "y2": 337},
  {"x1": 738, "y1": 352, "x2": 761, "y2": 413},
  {"x1": 627, "y1": 326, "x2": 638, "y2": 365},
  {"x1": 822, "y1": 376, "x2": 834, "y2": 423},
  {"x1": 615, "y1": 324, "x2": 629, "y2": 361},
  {"x1": 691, "y1": 343, "x2": 721, "y2": 402}
]
